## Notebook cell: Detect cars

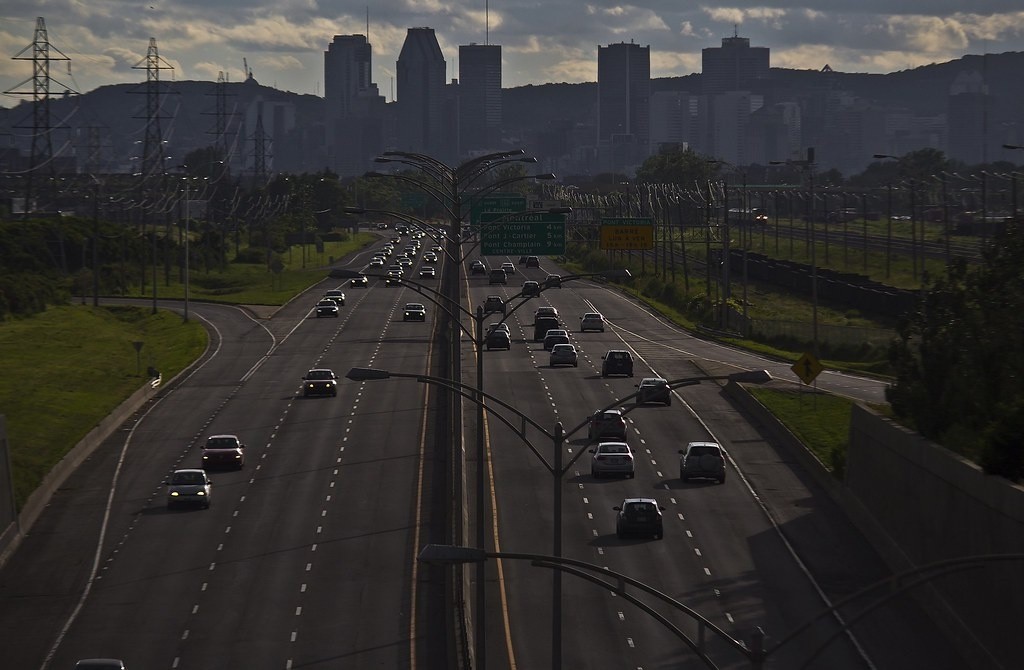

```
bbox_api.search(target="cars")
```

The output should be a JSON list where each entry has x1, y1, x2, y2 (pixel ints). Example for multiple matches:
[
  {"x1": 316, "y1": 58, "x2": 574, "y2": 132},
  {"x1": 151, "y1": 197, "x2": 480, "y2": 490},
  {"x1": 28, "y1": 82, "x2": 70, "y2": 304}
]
[
  {"x1": 588, "y1": 440, "x2": 636, "y2": 479},
  {"x1": 301, "y1": 368, "x2": 340, "y2": 399},
  {"x1": 167, "y1": 467, "x2": 214, "y2": 510},
  {"x1": 612, "y1": 496, "x2": 666, "y2": 541},
  {"x1": 586, "y1": 408, "x2": 629, "y2": 443},
  {"x1": 544, "y1": 328, "x2": 579, "y2": 368},
  {"x1": 634, "y1": 377, "x2": 672, "y2": 407},
  {"x1": 323, "y1": 289, "x2": 346, "y2": 306},
  {"x1": 600, "y1": 349, "x2": 635, "y2": 377},
  {"x1": 533, "y1": 305, "x2": 560, "y2": 324},
  {"x1": 401, "y1": 302, "x2": 427, "y2": 322},
  {"x1": 579, "y1": 312, "x2": 606, "y2": 333},
  {"x1": 484, "y1": 321, "x2": 513, "y2": 351},
  {"x1": 200, "y1": 434, "x2": 247, "y2": 471},
  {"x1": 315, "y1": 299, "x2": 339, "y2": 318},
  {"x1": 348, "y1": 222, "x2": 564, "y2": 314}
]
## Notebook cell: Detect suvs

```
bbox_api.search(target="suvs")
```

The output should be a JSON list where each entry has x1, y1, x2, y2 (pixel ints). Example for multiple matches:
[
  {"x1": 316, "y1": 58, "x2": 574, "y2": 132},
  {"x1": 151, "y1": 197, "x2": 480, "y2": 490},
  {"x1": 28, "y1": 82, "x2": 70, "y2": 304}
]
[
  {"x1": 532, "y1": 313, "x2": 562, "y2": 342},
  {"x1": 678, "y1": 440, "x2": 727, "y2": 485}
]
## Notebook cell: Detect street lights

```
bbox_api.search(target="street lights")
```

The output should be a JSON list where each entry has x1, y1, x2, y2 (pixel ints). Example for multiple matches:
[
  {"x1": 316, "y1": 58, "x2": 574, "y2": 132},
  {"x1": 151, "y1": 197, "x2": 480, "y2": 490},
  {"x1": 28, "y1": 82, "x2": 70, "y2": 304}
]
[
  {"x1": 345, "y1": 365, "x2": 775, "y2": 670},
  {"x1": 362, "y1": 148, "x2": 573, "y2": 287},
  {"x1": 872, "y1": 152, "x2": 925, "y2": 275},
  {"x1": 707, "y1": 158, "x2": 747, "y2": 248},
  {"x1": 767, "y1": 159, "x2": 815, "y2": 262},
  {"x1": 328, "y1": 262, "x2": 633, "y2": 670}
]
